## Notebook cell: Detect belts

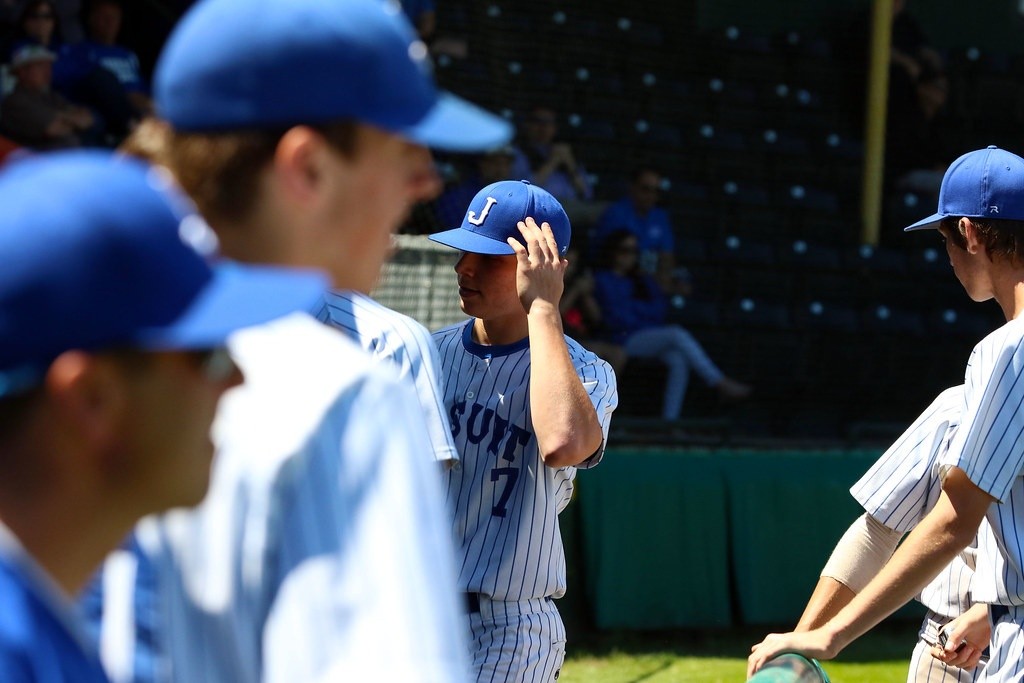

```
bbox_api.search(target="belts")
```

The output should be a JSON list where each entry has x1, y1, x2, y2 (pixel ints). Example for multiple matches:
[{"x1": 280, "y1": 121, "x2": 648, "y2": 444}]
[
  {"x1": 981, "y1": 645, "x2": 990, "y2": 656},
  {"x1": 991, "y1": 606, "x2": 1009, "y2": 626},
  {"x1": 461, "y1": 591, "x2": 552, "y2": 613}
]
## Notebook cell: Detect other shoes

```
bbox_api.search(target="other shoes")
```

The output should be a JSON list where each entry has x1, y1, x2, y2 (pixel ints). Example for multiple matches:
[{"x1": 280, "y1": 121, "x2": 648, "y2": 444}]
[
  {"x1": 719, "y1": 382, "x2": 754, "y2": 400},
  {"x1": 659, "y1": 430, "x2": 692, "y2": 445}
]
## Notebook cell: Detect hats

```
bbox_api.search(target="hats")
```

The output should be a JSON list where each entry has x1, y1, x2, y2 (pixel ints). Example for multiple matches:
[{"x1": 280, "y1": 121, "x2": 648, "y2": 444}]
[
  {"x1": 428, "y1": 180, "x2": 571, "y2": 256},
  {"x1": 13, "y1": 45, "x2": 58, "y2": 69},
  {"x1": 153, "y1": 0, "x2": 515, "y2": 153},
  {"x1": 904, "y1": 145, "x2": 1024, "y2": 231},
  {"x1": 0, "y1": 147, "x2": 325, "y2": 397}
]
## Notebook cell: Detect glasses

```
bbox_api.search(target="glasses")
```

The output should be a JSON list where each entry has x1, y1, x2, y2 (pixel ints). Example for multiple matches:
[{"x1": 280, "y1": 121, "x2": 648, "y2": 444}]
[
  {"x1": 30, "y1": 10, "x2": 51, "y2": 20},
  {"x1": 641, "y1": 184, "x2": 662, "y2": 196},
  {"x1": 617, "y1": 242, "x2": 639, "y2": 257}
]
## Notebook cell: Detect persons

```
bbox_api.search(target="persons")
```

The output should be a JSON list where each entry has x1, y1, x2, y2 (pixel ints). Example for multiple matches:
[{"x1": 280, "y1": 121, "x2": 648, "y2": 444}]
[
  {"x1": 749, "y1": 146, "x2": 1024, "y2": 683},
  {"x1": 512, "y1": 106, "x2": 752, "y2": 420},
  {"x1": 1, "y1": 0, "x2": 509, "y2": 683},
  {"x1": 428, "y1": 180, "x2": 619, "y2": 683},
  {"x1": 842, "y1": 1, "x2": 925, "y2": 169}
]
